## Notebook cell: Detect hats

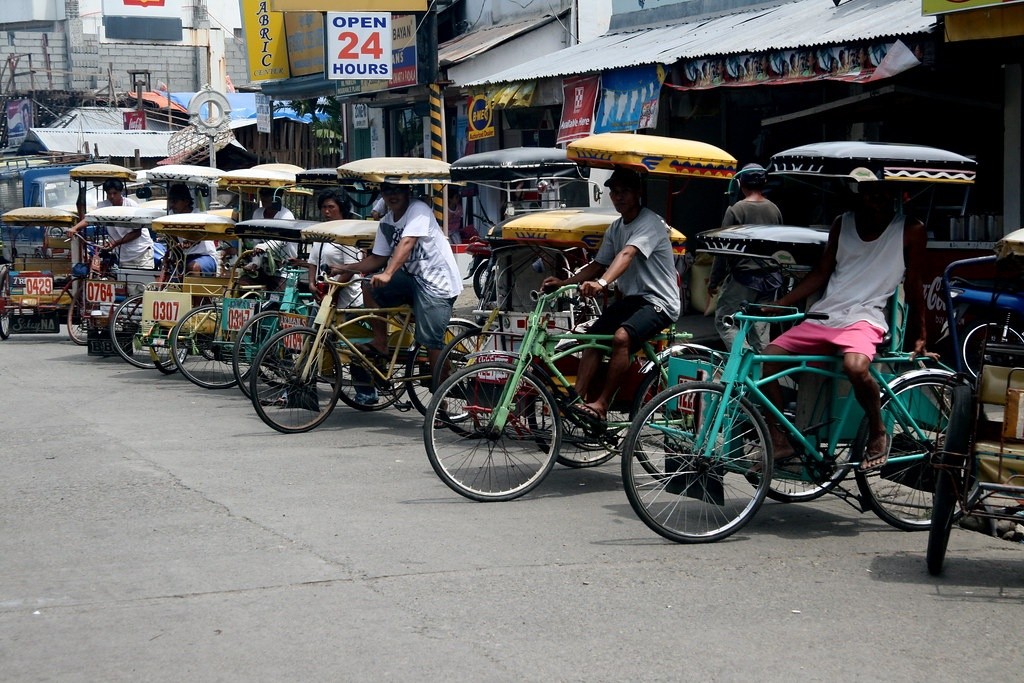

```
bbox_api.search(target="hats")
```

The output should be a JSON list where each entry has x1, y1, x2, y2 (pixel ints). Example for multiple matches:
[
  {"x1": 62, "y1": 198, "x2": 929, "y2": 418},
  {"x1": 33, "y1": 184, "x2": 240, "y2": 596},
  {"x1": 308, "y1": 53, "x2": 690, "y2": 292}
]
[
  {"x1": 165, "y1": 184, "x2": 195, "y2": 200},
  {"x1": 734, "y1": 163, "x2": 767, "y2": 185},
  {"x1": 604, "y1": 167, "x2": 640, "y2": 191}
]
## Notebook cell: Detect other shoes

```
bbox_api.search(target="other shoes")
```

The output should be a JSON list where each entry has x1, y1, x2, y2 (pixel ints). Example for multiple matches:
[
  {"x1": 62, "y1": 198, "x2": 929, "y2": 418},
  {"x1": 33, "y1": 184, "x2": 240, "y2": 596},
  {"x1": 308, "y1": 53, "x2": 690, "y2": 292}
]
[{"x1": 354, "y1": 391, "x2": 379, "y2": 405}]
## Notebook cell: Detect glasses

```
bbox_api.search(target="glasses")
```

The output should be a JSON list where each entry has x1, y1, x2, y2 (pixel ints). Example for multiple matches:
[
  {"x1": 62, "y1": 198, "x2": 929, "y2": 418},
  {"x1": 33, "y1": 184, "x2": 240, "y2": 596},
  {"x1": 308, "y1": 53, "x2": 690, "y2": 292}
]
[{"x1": 381, "y1": 186, "x2": 404, "y2": 195}]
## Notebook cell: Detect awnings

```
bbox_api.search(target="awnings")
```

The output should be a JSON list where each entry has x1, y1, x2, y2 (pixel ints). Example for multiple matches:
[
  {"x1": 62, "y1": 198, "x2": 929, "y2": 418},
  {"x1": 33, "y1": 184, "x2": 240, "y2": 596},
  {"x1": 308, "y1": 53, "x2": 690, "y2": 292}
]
[
  {"x1": 248, "y1": 107, "x2": 333, "y2": 124},
  {"x1": 16, "y1": 127, "x2": 247, "y2": 157}
]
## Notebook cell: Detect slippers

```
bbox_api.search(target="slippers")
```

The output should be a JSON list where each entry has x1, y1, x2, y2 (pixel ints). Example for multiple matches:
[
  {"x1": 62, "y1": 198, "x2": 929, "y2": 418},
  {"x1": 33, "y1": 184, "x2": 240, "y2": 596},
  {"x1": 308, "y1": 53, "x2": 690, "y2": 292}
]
[
  {"x1": 433, "y1": 401, "x2": 448, "y2": 429},
  {"x1": 350, "y1": 342, "x2": 390, "y2": 361},
  {"x1": 857, "y1": 433, "x2": 892, "y2": 473},
  {"x1": 574, "y1": 400, "x2": 607, "y2": 422},
  {"x1": 744, "y1": 453, "x2": 796, "y2": 477}
]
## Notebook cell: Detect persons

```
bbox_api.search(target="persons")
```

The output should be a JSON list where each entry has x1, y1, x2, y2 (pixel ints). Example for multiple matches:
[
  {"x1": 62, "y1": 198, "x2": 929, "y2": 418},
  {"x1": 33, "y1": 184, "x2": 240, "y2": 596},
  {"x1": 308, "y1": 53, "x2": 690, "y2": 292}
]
[
  {"x1": 66, "y1": 178, "x2": 155, "y2": 272},
  {"x1": 238, "y1": 183, "x2": 464, "y2": 437},
  {"x1": 498, "y1": 166, "x2": 681, "y2": 423},
  {"x1": 165, "y1": 184, "x2": 244, "y2": 308},
  {"x1": 707, "y1": 163, "x2": 784, "y2": 353},
  {"x1": 744, "y1": 179, "x2": 941, "y2": 473}
]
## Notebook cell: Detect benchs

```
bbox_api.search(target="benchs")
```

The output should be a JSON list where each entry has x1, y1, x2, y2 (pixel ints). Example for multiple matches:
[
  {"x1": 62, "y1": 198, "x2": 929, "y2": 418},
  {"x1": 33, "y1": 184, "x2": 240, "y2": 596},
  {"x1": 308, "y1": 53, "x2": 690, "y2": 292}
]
[
  {"x1": 471, "y1": 310, "x2": 576, "y2": 357},
  {"x1": 116, "y1": 269, "x2": 162, "y2": 292},
  {"x1": 981, "y1": 366, "x2": 1024, "y2": 421},
  {"x1": 185, "y1": 273, "x2": 242, "y2": 303},
  {"x1": 13, "y1": 257, "x2": 70, "y2": 282}
]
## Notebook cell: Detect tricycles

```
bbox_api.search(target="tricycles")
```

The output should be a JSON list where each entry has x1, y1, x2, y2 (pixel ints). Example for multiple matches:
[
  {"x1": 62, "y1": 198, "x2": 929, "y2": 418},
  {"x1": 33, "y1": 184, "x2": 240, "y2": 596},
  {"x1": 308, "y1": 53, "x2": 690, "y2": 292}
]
[
  {"x1": 0, "y1": 151, "x2": 743, "y2": 506},
  {"x1": 924, "y1": 250, "x2": 1023, "y2": 578},
  {"x1": 618, "y1": 141, "x2": 991, "y2": 542}
]
